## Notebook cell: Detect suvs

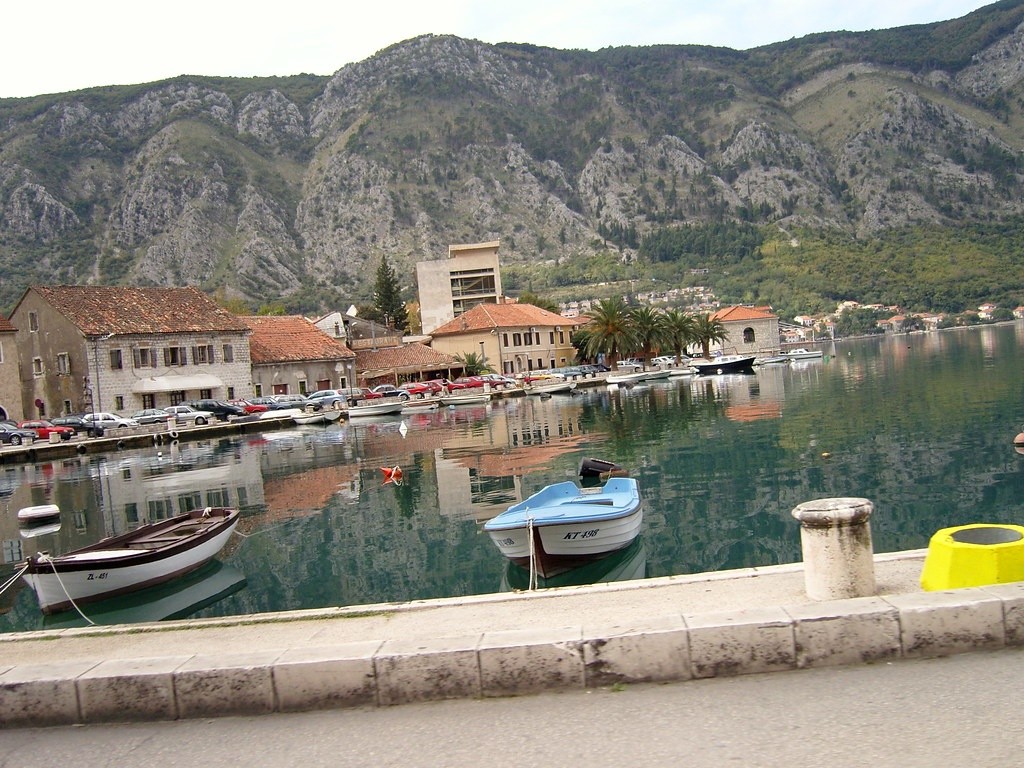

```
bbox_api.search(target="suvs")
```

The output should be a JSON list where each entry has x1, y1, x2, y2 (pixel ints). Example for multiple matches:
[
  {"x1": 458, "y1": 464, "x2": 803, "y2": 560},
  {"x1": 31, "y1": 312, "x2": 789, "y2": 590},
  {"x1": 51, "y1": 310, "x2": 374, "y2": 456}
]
[
  {"x1": 224, "y1": 399, "x2": 269, "y2": 414},
  {"x1": 339, "y1": 388, "x2": 366, "y2": 408},
  {"x1": 362, "y1": 388, "x2": 382, "y2": 399},
  {"x1": 179, "y1": 400, "x2": 249, "y2": 421}
]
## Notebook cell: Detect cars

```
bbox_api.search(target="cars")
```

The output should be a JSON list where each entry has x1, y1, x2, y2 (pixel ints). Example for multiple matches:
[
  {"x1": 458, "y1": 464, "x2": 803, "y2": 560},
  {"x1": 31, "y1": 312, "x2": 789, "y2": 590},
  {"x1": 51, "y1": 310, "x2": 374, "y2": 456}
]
[
  {"x1": 18, "y1": 419, "x2": 75, "y2": 440},
  {"x1": 0, "y1": 422, "x2": 40, "y2": 445},
  {"x1": 611, "y1": 361, "x2": 642, "y2": 373},
  {"x1": 373, "y1": 385, "x2": 411, "y2": 401},
  {"x1": 163, "y1": 405, "x2": 216, "y2": 425},
  {"x1": 308, "y1": 389, "x2": 348, "y2": 412},
  {"x1": 80, "y1": 413, "x2": 141, "y2": 429},
  {"x1": 130, "y1": 408, "x2": 180, "y2": 426},
  {"x1": 250, "y1": 394, "x2": 323, "y2": 413},
  {"x1": 399, "y1": 363, "x2": 611, "y2": 401},
  {"x1": 627, "y1": 355, "x2": 695, "y2": 369},
  {"x1": 51, "y1": 417, "x2": 104, "y2": 437}
]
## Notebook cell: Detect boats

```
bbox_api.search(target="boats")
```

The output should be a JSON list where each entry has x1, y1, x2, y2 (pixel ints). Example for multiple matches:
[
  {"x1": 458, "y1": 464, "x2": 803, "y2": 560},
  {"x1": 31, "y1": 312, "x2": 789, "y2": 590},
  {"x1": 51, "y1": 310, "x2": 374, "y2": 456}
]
[
  {"x1": 496, "y1": 533, "x2": 649, "y2": 594},
  {"x1": 11, "y1": 503, "x2": 243, "y2": 614},
  {"x1": 403, "y1": 402, "x2": 440, "y2": 413},
  {"x1": 687, "y1": 354, "x2": 758, "y2": 375},
  {"x1": 605, "y1": 369, "x2": 672, "y2": 392},
  {"x1": 32, "y1": 558, "x2": 247, "y2": 631},
  {"x1": 436, "y1": 393, "x2": 492, "y2": 406},
  {"x1": 18, "y1": 518, "x2": 63, "y2": 538},
  {"x1": 754, "y1": 356, "x2": 787, "y2": 368},
  {"x1": 665, "y1": 368, "x2": 699, "y2": 377},
  {"x1": 521, "y1": 381, "x2": 578, "y2": 397},
  {"x1": 346, "y1": 403, "x2": 403, "y2": 419},
  {"x1": 291, "y1": 413, "x2": 341, "y2": 427},
  {"x1": 478, "y1": 458, "x2": 650, "y2": 576},
  {"x1": 17, "y1": 503, "x2": 60, "y2": 527},
  {"x1": 778, "y1": 348, "x2": 823, "y2": 359}
]
